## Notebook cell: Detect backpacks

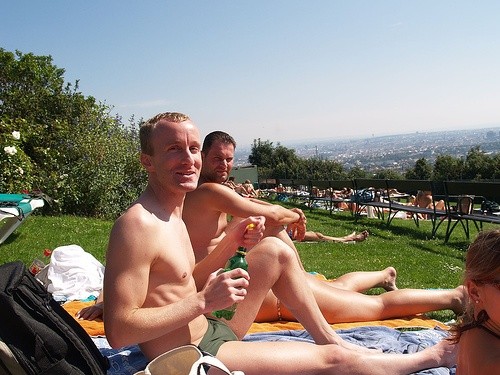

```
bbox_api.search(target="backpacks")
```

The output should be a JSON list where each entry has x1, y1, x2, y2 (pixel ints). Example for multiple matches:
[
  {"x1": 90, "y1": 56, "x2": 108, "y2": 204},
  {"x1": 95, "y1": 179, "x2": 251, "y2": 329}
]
[{"x1": 0, "y1": 261, "x2": 107, "y2": 375}]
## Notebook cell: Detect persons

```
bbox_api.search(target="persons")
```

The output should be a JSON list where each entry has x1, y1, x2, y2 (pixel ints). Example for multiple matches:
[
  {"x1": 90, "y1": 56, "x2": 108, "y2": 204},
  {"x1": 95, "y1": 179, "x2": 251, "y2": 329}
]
[
  {"x1": 446, "y1": 230, "x2": 500, "y2": 375},
  {"x1": 182, "y1": 130, "x2": 307, "y2": 271},
  {"x1": 106, "y1": 112, "x2": 462, "y2": 375},
  {"x1": 76, "y1": 175, "x2": 472, "y2": 322}
]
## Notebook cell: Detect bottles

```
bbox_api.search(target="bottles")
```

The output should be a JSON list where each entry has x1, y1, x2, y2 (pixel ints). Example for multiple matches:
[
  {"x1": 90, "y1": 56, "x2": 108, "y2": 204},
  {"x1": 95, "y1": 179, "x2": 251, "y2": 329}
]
[
  {"x1": 27, "y1": 248, "x2": 52, "y2": 278},
  {"x1": 210, "y1": 245, "x2": 249, "y2": 321}
]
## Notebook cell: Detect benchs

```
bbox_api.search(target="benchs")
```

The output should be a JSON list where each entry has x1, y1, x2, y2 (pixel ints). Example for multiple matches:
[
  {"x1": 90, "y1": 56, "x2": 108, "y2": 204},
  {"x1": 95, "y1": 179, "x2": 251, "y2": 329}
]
[{"x1": 259, "y1": 190, "x2": 500, "y2": 244}]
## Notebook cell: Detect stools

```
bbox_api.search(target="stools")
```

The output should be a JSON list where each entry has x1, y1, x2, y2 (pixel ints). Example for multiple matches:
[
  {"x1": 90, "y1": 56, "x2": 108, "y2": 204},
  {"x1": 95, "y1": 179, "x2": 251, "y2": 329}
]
[{"x1": 0, "y1": 194, "x2": 44, "y2": 244}]
